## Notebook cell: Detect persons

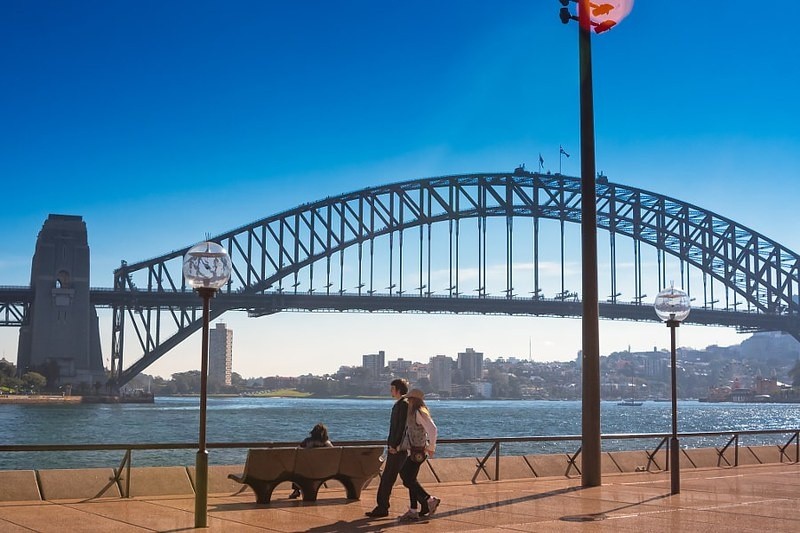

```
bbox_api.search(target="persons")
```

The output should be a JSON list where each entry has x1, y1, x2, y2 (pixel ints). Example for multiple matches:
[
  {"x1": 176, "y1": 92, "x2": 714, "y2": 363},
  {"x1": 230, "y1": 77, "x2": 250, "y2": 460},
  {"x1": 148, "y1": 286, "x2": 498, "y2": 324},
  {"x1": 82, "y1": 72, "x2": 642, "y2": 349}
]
[
  {"x1": 289, "y1": 424, "x2": 333, "y2": 500},
  {"x1": 364, "y1": 380, "x2": 441, "y2": 521}
]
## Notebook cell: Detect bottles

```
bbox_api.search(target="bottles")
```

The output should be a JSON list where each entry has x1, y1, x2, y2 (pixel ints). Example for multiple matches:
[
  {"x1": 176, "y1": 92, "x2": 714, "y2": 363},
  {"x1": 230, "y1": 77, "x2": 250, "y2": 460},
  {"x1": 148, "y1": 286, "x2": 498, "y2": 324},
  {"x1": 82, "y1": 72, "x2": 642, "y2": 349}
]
[{"x1": 379, "y1": 448, "x2": 388, "y2": 462}]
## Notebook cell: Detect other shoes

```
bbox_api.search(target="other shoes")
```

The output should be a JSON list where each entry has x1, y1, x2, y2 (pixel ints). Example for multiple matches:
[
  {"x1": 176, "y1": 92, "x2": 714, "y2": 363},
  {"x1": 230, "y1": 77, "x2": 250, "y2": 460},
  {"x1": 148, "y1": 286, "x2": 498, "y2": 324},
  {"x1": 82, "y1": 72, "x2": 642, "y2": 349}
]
[
  {"x1": 419, "y1": 500, "x2": 429, "y2": 515},
  {"x1": 365, "y1": 506, "x2": 388, "y2": 517},
  {"x1": 289, "y1": 490, "x2": 300, "y2": 499},
  {"x1": 428, "y1": 496, "x2": 441, "y2": 517},
  {"x1": 398, "y1": 505, "x2": 420, "y2": 522}
]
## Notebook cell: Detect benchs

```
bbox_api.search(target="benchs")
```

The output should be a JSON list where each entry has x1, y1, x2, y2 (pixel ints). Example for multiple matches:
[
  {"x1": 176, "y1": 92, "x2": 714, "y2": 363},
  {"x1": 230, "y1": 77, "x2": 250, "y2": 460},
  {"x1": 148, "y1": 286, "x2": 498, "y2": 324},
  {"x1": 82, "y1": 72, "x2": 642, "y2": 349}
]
[{"x1": 228, "y1": 446, "x2": 384, "y2": 504}]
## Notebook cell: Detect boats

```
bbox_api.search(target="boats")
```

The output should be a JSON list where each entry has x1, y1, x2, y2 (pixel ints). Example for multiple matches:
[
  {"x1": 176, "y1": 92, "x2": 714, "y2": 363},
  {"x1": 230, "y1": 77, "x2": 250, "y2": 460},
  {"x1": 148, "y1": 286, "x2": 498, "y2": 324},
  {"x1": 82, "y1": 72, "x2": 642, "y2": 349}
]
[
  {"x1": 653, "y1": 399, "x2": 672, "y2": 403},
  {"x1": 617, "y1": 399, "x2": 643, "y2": 406}
]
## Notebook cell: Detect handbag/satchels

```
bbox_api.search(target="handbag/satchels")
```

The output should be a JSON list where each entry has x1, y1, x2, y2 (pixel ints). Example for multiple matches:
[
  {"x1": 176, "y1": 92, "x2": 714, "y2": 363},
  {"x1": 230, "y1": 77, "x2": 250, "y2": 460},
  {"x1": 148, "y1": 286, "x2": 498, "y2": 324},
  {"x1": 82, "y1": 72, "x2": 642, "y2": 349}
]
[{"x1": 410, "y1": 446, "x2": 425, "y2": 463}]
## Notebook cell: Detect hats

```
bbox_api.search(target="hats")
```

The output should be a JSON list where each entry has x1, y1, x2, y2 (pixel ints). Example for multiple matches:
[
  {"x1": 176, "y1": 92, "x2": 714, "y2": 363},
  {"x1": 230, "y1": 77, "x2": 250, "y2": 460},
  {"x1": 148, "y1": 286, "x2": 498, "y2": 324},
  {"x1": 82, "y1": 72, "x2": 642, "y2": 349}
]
[{"x1": 404, "y1": 389, "x2": 424, "y2": 400}]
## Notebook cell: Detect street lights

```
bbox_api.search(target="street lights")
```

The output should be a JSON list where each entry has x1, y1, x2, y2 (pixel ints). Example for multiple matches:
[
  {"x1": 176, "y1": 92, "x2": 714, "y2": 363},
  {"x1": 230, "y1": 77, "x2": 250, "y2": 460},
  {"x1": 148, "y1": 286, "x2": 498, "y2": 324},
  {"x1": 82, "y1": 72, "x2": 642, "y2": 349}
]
[
  {"x1": 182, "y1": 241, "x2": 233, "y2": 528},
  {"x1": 559, "y1": 1, "x2": 616, "y2": 489},
  {"x1": 653, "y1": 288, "x2": 690, "y2": 495}
]
